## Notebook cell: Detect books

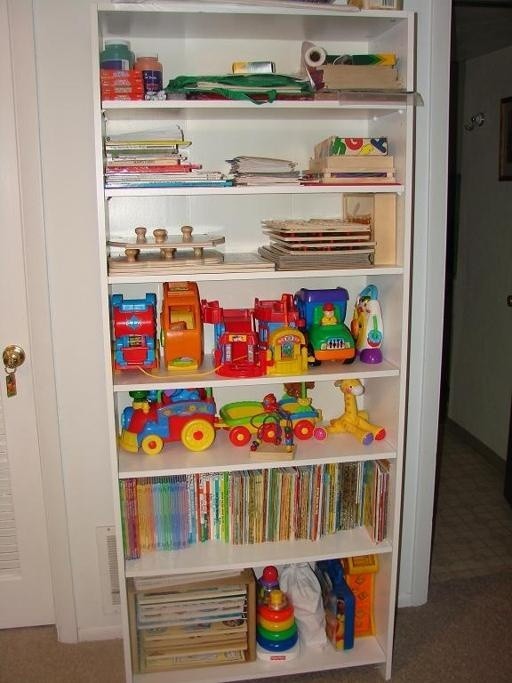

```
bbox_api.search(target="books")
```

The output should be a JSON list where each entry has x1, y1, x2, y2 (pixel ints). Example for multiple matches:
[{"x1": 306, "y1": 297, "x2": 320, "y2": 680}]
[
  {"x1": 226, "y1": 156, "x2": 303, "y2": 186},
  {"x1": 104, "y1": 126, "x2": 232, "y2": 189},
  {"x1": 259, "y1": 192, "x2": 396, "y2": 269},
  {"x1": 303, "y1": 136, "x2": 396, "y2": 186},
  {"x1": 120, "y1": 458, "x2": 394, "y2": 562},
  {"x1": 131, "y1": 566, "x2": 251, "y2": 674}
]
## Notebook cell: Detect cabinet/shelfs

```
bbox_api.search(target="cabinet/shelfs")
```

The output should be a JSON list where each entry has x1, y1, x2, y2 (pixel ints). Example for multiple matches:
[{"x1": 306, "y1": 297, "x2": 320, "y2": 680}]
[{"x1": 89, "y1": 2, "x2": 415, "y2": 683}]
